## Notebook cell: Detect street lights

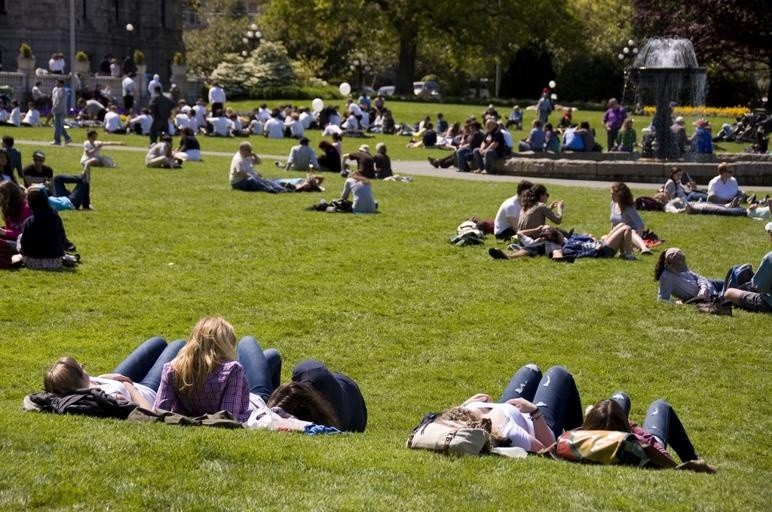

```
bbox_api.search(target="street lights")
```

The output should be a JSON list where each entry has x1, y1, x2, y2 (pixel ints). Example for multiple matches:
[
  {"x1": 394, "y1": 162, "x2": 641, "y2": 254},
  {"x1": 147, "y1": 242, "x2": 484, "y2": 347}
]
[
  {"x1": 123, "y1": 23, "x2": 137, "y2": 78},
  {"x1": 242, "y1": 22, "x2": 263, "y2": 59},
  {"x1": 617, "y1": 40, "x2": 639, "y2": 69},
  {"x1": 350, "y1": 53, "x2": 371, "y2": 95},
  {"x1": 547, "y1": 80, "x2": 558, "y2": 106}
]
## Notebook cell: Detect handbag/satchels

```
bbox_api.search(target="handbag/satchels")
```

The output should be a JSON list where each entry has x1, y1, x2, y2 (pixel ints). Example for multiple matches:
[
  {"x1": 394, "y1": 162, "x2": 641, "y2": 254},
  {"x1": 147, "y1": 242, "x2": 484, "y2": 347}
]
[
  {"x1": 406, "y1": 419, "x2": 491, "y2": 459},
  {"x1": 555, "y1": 424, "x2": 651, "y2": 467},
  {"x1": 722, "y1": 263, "x2": 756, "y2": 293}
]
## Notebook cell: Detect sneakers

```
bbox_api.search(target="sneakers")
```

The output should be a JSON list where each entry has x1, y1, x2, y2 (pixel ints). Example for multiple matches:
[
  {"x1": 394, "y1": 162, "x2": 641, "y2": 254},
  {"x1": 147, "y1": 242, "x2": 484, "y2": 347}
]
[
  {"x1": 730, "y1": 196, "x2": 742, "y2": 208},
  {"x1": 760, "y1": 194, "x2": 769, "y2": 207},
  {"x1": 275, "y1": 160, "x2": 284, "y2": 167},
  {"x1": 488, "y1": 247, "x2": 508, "y2": 260},
  {"x1": 747, "y1": 193, "x2": 756, "y2": 205},
  {"x1": 48, "y1": 137, "x2": 72, "y2": 145},
  {"x1": 427, "y1": 156, "x2": 491, "y2": 174},
  {"x1": 82, "y1": 163, "x2": 91, "y2": 182},
  {"x1": 552, "y1": 255, "x2": 575, "y2": 264},
  {"x1": 696, "y1": 297, "x2": 734, "y2": 318},
  {"x1": 83, "y1": 205, "x2": 95, "y2": 211}
]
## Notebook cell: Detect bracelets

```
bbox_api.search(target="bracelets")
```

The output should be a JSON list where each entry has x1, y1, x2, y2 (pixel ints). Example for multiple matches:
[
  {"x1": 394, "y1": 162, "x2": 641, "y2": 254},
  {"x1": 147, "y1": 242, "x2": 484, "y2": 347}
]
[
  {"x1": 530, "y1": 413, "x2": 544, "y2": 421},
  {"x1": 528, "y1": 407, "x2": 540, "y2": 416}
]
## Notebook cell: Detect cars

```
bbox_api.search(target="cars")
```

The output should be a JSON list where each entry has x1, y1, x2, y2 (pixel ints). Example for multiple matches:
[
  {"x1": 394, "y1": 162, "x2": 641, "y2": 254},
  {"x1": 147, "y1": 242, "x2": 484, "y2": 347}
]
[{"x1": 354, "y1": 78, "x2": 493, "y2": 98}]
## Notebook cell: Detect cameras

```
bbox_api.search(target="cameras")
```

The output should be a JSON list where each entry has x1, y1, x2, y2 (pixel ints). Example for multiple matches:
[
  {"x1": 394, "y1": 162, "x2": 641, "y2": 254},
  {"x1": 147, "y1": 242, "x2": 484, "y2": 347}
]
[{"x1": 553, "y1": 202, "x2": 559, "y2": 207}]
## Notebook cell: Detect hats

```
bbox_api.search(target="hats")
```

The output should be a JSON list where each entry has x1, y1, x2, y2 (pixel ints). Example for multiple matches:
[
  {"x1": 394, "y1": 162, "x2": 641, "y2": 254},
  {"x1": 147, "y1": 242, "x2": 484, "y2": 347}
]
[
  {"x1": 27, "y1": 180, "x2": 46, "y2": 201},
  {"x1": 543, "y1": 87, "x2": 550, "y2": 94},
  {"x1": 31, "y1": 150, "x2": 45, "y2": 158},
  {"x1": 299, "y1": 136, "x2": 311, "y2": 144},
  {"x1": 563, "y1": 111, "x2": 570, "y2": 120},
  {"x1": 668, "y1": 165, "x2": 680, "y2": 177},
  {"x1": 606, "y1": 98, "x2": 617, "y2": 107},
  {"x1": 692, "y1": 120, "x2": 705, "y2": 127},
  {"x1": 675, "y1": 116, "x2": 686, "y2": 125}
]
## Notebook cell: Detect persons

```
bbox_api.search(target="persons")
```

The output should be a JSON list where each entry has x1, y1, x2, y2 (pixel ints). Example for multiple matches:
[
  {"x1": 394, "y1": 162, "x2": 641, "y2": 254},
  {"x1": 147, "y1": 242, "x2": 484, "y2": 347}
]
[
  {"x1": 541, "y1": 222, "x2": 639, "y2": 260},
  {"x1": 144, "y1": 135, "x2": 182, "y2": 170},
  {"x1": 265, "y1": 358, "x2": 367, "y2": 435},
  {"x1": 320, "y1": 168, "x2": 378, "y2": 213},
  {"x1": 653, "y1": 244, "x2": 754, "y2": 308},
  {"x1": 436, "y1": 362, "x2": 584, "y2": 461},
  {"x1": 721, "y1": 221, "x2": 772, "y2": 315},
  {"x1": 274, "y1": 139, "x2": 319, "y2": 173},
  {"x1": 316, "y1": 141, "x2": 342, "y2": 173},
  {"x1": 515, "y1": 183, "x2": 565, "y2": 232},
  {"x1": 583, "y1": 389, "x2": 718, "y2": 474},
  {"x1": 0, "y1": 52, "x2": 412, "y2": 145},
  {"x1": 600, "y1": 181, "x2": 654, "y2": 257},
  {"x1": 372, "y1": 143, "x2": 393, "y2": 179},
  {"x1": 492, "y1": 179, "x2": 534, "y2": 240},
  {"x1": 413, "y1": 81, "x2": 772, "y2": 177},
  {"x1": 653, "y1": 161, "x2": 771, "y2": 222},
  {"x1": 339, "y1": 145, "x2": 377, "y2": 181},
  {"x1": 228, "y1": 142, "x2": 297, "y2": 194},
  {"x1": 170, "y1": 126, "x2": 202, "y2": 162},
  {"x1": 273, "y1": 171, "x2": 325, "y2": 192},
  {"x1": 154, "y1": 311, "x2": 284, "y2": 429},
  {"x1": 42, "y1": 335, "x2": 188, "y2": 417},
  {"x1": 331, "y1": 133, "x2": 343, "y2": 154},
  {"x1": 0, "y1": 130, "x2": 96, "y2": 274},
  {"x1": 487, "y1": 223, "x2": 574, "y2": 265},
  {"x1": 79, "y1": 130, "x2": 127, "y2": 168}
]
